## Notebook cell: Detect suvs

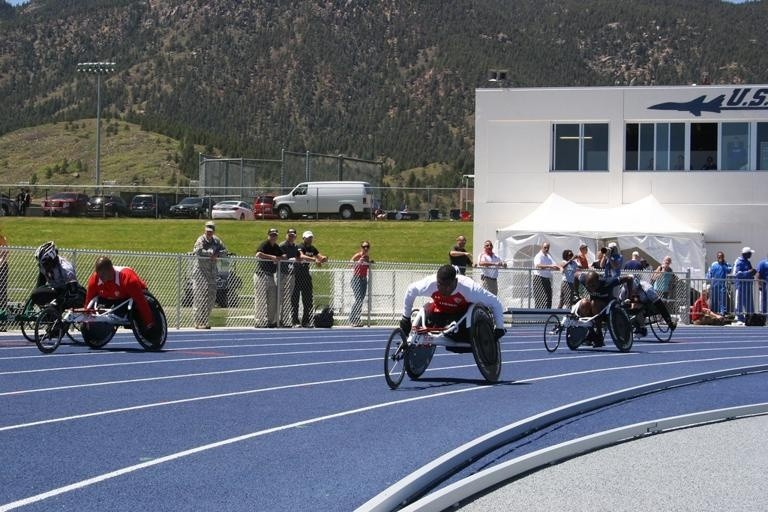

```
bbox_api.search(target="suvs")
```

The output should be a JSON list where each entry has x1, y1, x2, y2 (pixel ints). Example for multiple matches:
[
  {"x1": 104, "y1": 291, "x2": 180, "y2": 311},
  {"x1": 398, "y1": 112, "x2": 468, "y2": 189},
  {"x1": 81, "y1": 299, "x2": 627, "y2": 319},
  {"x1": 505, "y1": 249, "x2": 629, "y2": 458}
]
[
  {"x1": 40, "y1": 191, "x2": 89, "y2": 217},
  {"x1": 254, "y1": 194, "x2": 280, "y2": 220},
  {"x1": 128, "y1": 195, "x2": 173, "y2": 219}
]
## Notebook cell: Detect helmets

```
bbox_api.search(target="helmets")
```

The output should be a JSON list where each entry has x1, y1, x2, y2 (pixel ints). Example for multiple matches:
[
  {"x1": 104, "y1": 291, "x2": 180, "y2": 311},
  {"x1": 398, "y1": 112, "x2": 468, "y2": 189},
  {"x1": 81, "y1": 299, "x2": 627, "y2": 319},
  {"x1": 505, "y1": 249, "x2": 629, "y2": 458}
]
[{"x1": 35, "y1": 240, "x2": 57, "y2": 263}]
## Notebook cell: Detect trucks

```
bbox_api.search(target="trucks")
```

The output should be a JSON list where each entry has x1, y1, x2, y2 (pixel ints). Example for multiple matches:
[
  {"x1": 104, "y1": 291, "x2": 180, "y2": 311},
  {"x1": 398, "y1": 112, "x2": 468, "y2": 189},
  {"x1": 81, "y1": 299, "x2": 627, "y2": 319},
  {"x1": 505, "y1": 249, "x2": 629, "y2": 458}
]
[{"x1": 273, "y1": 181, "x2": 374, "y2": 219}]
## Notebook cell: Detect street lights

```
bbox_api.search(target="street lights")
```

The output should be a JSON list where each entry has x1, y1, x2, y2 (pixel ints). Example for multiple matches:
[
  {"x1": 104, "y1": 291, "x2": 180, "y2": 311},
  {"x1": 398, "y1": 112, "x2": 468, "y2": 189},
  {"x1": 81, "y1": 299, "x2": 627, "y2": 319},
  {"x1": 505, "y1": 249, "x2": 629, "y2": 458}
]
[{"x1": 76, "y1": 62, "x2": 116, "y2": 196}]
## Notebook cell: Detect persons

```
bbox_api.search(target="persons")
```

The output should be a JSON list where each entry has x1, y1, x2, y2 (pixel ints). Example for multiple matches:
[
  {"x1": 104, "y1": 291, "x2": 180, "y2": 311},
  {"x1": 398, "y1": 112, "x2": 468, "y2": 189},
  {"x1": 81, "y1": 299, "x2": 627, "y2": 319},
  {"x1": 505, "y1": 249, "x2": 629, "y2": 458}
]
[
  {"x1": 191, "y1": 221, "x2": 228, "y2": 329},
  {"x1": 83, "y1": 255, "x2": 154, "y2": 329},
  {"x1": 253, "y1": 229, "x2": 286, "y2": 328},
  {"x1": 290, "y1": 230, "x2": 328, "y2": 328},
  {"x1": 400, "y1": 264, "x2": 505, "y2": 339},
  {"x1": 530, "y1": 242, "x2": 768, "y2": 343},
  {"x1": 479, "y1": 240, "x2": 507, "y2": 296},
  {"x1": 348, "y1": 240, "x2": 373, "y2": 327},
  {"x1": 449, "y1": 236, "x2": 477, "y2": 275},
  {"x1": 0, "y1": 228, "x2": 10, "y2": 332},
  {"x1": 276, "y1": 228, "x2": 302, "y2": 327},
  {"x1": 25, "y1": 240, "x2": 78, "y2": 316}
]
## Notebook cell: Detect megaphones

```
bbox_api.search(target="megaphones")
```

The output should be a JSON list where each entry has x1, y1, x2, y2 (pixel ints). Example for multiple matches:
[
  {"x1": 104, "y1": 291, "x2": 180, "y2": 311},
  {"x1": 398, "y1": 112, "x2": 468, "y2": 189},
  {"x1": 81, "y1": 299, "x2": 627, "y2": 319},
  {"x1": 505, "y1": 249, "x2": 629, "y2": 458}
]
[
  {"x1": 498, "y1": 70, "x2": 508, "y2": 82},
  {"x1": 487, "y1": 69, "x2": 498, "y2": 81}
]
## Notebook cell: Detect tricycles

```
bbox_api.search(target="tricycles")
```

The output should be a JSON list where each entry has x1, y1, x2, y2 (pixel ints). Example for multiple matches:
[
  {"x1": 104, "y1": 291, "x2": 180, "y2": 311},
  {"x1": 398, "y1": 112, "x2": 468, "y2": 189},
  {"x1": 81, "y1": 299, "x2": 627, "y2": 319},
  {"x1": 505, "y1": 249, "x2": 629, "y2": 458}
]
[
  {"x1": 1, "y1": 283, "x2": 88, "y2": 343},
  {"x1": 544, "y1": 295, "x2": 634, "y2": 354},
  {"x1": 588, "y1": 296, "x2": 675, "y2": 345},
  {"x1": 383, "y1": 300, "x2": 502, "y2": 391},
  {"x1": 34, "y1": 285, "x2": 168, "y2": 354}
]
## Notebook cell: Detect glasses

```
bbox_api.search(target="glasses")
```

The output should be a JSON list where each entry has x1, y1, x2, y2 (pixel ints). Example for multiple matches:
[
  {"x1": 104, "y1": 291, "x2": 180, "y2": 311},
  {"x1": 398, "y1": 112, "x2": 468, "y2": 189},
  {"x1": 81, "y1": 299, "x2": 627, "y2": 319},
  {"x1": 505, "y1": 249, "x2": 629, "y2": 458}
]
[{"x1": 361, "y1": 244, "x2": 369, "y2": 248}]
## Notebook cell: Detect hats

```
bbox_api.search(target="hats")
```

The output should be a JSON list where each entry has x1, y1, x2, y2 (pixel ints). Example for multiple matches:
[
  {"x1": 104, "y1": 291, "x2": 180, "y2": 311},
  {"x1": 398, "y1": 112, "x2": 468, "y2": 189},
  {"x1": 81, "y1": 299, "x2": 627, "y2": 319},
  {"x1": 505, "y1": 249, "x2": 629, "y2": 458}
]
[
  {"x1": 205, "y1": 221, "x2": 215, "y2": 232},
  {"x1": 741, "y1": 246, "x2": 755, "y2": 254},
  {"x1": 268, "y1": 228, "x2": 314, "y2": 239}
]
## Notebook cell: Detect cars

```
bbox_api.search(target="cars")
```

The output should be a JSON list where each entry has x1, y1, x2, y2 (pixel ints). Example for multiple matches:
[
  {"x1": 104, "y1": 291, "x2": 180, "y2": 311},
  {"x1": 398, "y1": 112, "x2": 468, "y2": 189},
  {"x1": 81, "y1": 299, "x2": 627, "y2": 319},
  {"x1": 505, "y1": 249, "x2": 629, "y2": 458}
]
[
  {"x1": 212, "y1": 201, "x2": 255, "y2": 221},
  {"x1": 373, "y1": 208, "x2": 420, "y2": 220},
  {"x1": 169, "y1": 197, "x2": 217, "y2": 219},
  {"x1": 0, "y1": 190, "x2": 18, "y2": 217},
  {"x1": 83, "y1": 195, "x2": 129, "y2": 219}
]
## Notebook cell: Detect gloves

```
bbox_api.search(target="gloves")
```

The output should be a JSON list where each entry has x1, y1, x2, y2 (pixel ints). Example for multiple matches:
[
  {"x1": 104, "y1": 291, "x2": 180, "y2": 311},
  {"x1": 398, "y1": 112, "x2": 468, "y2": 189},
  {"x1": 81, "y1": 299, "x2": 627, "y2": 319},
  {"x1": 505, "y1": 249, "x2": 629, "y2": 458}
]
[
  {"x1": 494, "y1": 328, "x2": 504, "y2": 341},
  {"x1": 571, "y1": 289, "x2": 581, "y2": 303},
  {"x1": 400, "y1": 315, "x2": 412, "y2": 337}
]
